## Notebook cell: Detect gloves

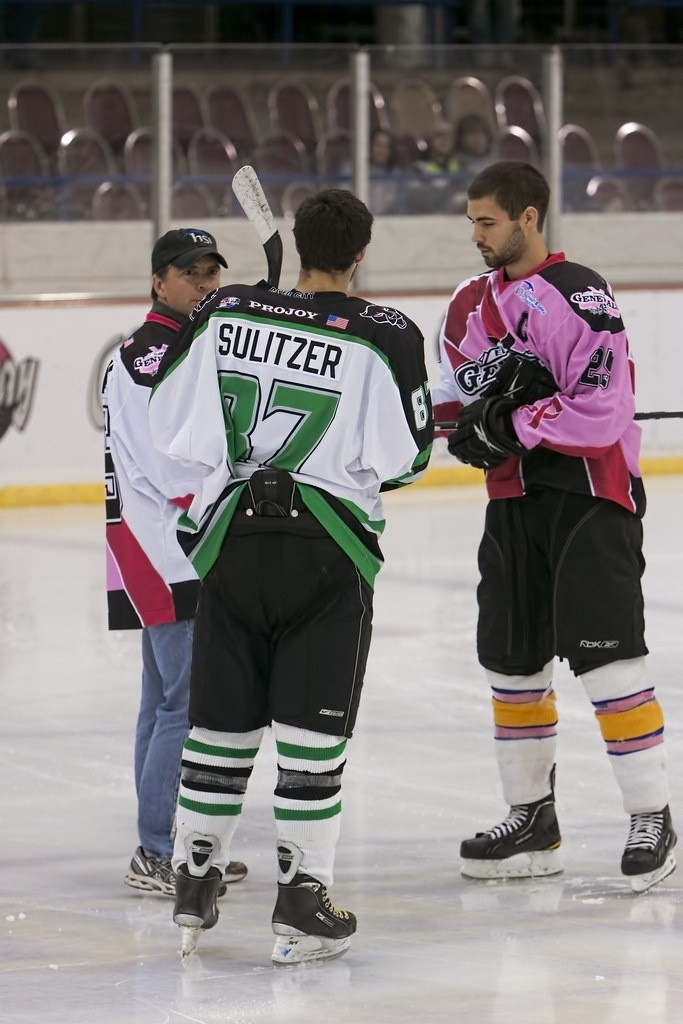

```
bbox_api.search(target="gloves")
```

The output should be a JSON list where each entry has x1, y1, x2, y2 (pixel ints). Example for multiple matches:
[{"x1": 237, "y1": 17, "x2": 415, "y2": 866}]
[
  {"x1": 448, "y1": 397, "x2": 529, "y2": 470},
  {"x1": 482, "y1": 356, "x2": 562, "y2": 406}
]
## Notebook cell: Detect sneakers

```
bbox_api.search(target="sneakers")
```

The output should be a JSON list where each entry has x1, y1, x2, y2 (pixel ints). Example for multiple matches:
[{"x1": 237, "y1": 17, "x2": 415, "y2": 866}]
[
  {"x1": 124, "y1": 845, "x2": 226, "y2": 896},
  {"x1": 460, "y1": 762, "x2": 564, "y2": 879},
  {"x1": 173, "y1": 831, "x2": 222, "y2": 958},
  {"x1": 622, "y1": 804, "x2": 677, "y2": 893},
  {"x1": 272, "y1": 840, "x2": 357, "y2": 964},
  {"x1": 222, "y1": 861, "x2": 248, "y2": 883}
]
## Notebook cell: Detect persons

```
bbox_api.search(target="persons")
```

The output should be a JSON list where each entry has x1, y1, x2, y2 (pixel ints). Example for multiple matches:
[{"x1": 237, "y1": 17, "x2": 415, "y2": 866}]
[
  {"x1": 429, "y1": 160, "x2": 683, "y2": 877},
  {"x1": 100, "y1": 228, "x2": 249, "y2": 900},
  {"x1": 148, "y1": 188, "x2": 435, "y2": 939},
  {"x1": 337, "y1": 112, "x2": 508, "y2": 214}
]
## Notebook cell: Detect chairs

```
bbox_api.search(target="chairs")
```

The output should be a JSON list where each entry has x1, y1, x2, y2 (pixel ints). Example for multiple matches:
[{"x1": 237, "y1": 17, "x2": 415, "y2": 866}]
[{"x1": 0, "y1": 74, "x2": 683, "y2": 223}]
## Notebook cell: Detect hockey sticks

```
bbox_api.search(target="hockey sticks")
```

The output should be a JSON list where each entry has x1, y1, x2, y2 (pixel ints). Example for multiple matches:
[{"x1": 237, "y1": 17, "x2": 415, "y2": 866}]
[
  {"x1": 231, "y1": 164, "x2": 283, "y2": 290},
  {"x1": 433, "y1": 411, "x2": 683, "y2": 432}
]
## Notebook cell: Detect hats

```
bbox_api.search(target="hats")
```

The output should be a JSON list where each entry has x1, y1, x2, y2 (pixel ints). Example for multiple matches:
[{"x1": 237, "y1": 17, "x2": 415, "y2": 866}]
[{"x1": 152, "y1": 229, "x2": 228, "y2": 276}]
